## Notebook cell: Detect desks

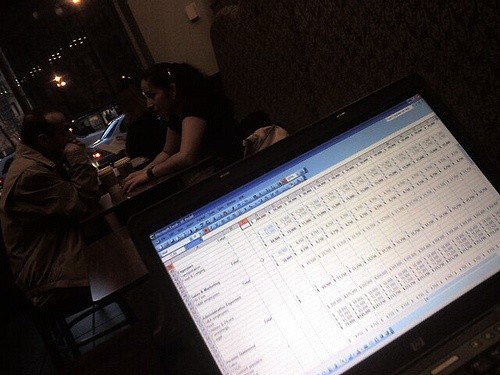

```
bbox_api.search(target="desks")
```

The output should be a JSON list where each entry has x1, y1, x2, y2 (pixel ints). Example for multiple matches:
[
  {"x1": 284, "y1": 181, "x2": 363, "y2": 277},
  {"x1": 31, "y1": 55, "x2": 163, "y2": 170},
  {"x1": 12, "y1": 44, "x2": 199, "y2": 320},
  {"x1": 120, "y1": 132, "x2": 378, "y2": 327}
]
[{"x1": 79, "y1": 152, "x2": 212, "y2": 224}]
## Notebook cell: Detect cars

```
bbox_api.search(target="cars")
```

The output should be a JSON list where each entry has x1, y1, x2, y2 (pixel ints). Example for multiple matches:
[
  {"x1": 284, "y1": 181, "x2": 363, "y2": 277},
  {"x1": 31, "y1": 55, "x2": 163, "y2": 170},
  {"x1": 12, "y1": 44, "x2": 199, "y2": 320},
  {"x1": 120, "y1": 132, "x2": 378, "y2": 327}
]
[{"x1": 87, "y1": 113, "x2": 127, "y2": 155}]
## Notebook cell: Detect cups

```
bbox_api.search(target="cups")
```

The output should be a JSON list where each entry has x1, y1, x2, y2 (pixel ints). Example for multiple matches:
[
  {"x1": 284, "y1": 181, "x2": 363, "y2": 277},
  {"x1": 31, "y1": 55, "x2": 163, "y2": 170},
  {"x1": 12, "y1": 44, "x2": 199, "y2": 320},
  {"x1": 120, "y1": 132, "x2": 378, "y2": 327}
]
[
  {"x1": 97, "y1": 165, "x2": 118, "y2": 187},
  {"x1": 113, "y1": 156, "x2": 134, "y2": 177}
]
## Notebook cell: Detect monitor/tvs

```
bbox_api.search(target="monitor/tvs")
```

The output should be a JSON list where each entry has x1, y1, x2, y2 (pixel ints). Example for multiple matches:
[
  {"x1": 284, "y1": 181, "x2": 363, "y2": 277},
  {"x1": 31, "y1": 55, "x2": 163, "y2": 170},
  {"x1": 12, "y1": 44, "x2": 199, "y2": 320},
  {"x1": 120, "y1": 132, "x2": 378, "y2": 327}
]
[{"x1": 123, "y1": 70, "x2": 500, "y2": 375}]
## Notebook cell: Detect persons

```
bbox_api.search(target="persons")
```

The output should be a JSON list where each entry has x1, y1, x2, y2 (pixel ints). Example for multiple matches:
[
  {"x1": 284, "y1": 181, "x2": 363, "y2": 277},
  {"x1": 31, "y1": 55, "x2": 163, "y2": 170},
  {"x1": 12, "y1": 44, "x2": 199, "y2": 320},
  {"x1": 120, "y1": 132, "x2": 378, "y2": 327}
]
[
  {"x1": 121, "y1": 66, "x2": 166, "y2": 159},
  {"x1": 122, "y1": 62, "x2": 242, "y2": 196},
  {"x1": 0, "y1": 108, "x2": 102, "y2": 295}
]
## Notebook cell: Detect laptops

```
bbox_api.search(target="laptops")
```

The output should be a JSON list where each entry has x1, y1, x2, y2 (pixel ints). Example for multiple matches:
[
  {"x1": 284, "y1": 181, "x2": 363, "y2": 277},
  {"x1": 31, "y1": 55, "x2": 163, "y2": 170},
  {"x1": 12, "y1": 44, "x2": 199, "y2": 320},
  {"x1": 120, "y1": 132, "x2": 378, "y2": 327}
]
[{"x1": 85, "y1": 113, "x2": 124, "y2": 153}]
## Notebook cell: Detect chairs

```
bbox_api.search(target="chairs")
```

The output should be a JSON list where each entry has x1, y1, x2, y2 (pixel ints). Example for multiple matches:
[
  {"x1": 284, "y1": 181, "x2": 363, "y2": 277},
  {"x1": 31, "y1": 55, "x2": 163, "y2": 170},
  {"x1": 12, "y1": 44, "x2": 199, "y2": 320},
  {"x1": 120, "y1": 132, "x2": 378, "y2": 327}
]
[{"x1": 1, "y1": 266, "x2": 135, "y2": 359}]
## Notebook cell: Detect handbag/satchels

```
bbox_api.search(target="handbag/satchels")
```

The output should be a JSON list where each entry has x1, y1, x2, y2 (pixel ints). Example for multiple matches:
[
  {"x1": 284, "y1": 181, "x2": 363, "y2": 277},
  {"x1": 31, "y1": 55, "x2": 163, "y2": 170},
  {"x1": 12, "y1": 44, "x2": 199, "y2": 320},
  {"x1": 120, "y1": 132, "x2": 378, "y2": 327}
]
[{"x1": 243, "y1": 124, "x2": 290, "y2": 159}]
